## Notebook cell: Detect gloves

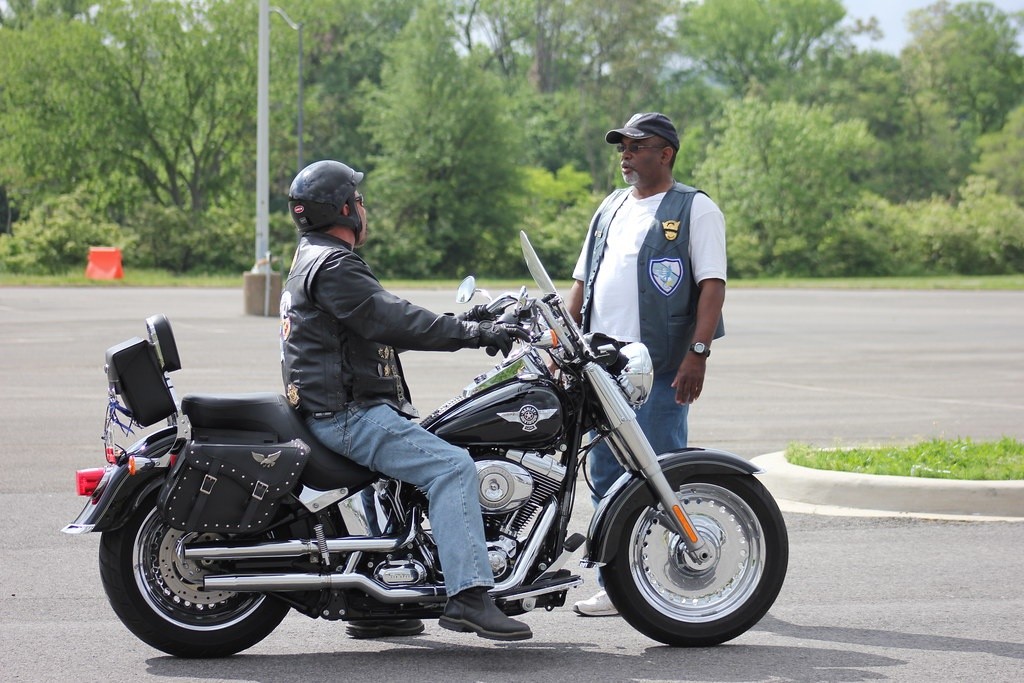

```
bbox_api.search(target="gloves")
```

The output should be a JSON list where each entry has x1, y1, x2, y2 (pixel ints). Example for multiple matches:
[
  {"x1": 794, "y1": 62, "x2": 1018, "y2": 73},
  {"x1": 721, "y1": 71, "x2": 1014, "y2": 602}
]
[{"x1": 479, "y1": 320, "x2": 533, "y2": 358}]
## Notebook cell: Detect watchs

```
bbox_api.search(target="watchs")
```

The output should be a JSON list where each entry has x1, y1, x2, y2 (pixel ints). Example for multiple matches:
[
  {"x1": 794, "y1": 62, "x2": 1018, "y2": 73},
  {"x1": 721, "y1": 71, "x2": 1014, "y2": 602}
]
[{"x1": 689, "y1": 342, "x2": 712, "y2": 356}]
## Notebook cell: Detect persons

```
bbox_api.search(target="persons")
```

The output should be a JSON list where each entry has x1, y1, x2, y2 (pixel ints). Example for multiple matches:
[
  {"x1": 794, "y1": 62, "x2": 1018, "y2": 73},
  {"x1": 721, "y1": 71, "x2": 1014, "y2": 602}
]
[
  {"x1": 279, "y1": 160, "x2": 532, "y2": 640},
  {"x1": 550, "y1": 113, "x2": 730, "y2": 618}
]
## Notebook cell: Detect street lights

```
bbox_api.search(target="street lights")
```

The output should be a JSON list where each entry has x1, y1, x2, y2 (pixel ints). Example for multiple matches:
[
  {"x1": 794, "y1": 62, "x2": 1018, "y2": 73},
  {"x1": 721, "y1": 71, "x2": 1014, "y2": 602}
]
[{"x1": 268, "y1": 7, "x2": 301, "y2": 247}]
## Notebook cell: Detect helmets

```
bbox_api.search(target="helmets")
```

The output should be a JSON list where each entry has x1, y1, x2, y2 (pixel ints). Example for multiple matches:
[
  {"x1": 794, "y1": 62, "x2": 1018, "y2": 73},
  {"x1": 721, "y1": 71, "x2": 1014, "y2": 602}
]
[{"x1": 288, "y1": 160, "x2": 364, "y2": 234}]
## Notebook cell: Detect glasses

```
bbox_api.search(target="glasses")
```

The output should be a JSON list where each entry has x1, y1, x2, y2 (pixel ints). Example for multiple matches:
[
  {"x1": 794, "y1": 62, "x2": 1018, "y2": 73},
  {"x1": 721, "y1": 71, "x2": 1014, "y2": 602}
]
[
  {"x1": 616, "y1": 145, "x2": 666, "y2": 153},
  {"x1": 355, "y1": 194, "x2": 364, "y2": 205}
]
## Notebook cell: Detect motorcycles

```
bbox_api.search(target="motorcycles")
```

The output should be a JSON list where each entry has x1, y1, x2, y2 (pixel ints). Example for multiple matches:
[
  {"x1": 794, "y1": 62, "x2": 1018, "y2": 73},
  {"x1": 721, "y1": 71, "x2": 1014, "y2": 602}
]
[{"x1": 60, "y1": 228, "x2": 789, "y2": 658}]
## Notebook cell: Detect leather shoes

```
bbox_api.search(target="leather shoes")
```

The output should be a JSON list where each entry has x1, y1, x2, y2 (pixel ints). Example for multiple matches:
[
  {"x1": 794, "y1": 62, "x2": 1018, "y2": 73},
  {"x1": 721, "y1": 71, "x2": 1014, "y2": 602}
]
[
  {"x1": 438, "y1": 586, "x2": 533, "y2": 640},
  {"x1": 345, "y1": 619, "x2": 424, "y2": 639}
]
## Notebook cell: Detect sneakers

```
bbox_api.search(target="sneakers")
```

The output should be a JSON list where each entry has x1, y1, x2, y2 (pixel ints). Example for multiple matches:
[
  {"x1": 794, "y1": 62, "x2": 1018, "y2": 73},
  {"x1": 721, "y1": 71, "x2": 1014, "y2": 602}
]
[{"x1": 573, "y1": 588, "x2": 619, "y2": 616}]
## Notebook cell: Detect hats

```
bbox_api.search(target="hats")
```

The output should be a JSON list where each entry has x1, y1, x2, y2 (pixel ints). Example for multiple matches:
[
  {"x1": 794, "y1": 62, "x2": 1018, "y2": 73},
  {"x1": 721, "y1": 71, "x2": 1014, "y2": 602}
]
[{"x1": 605, "y1": 112, "x2": 680, "y2": 152}]
